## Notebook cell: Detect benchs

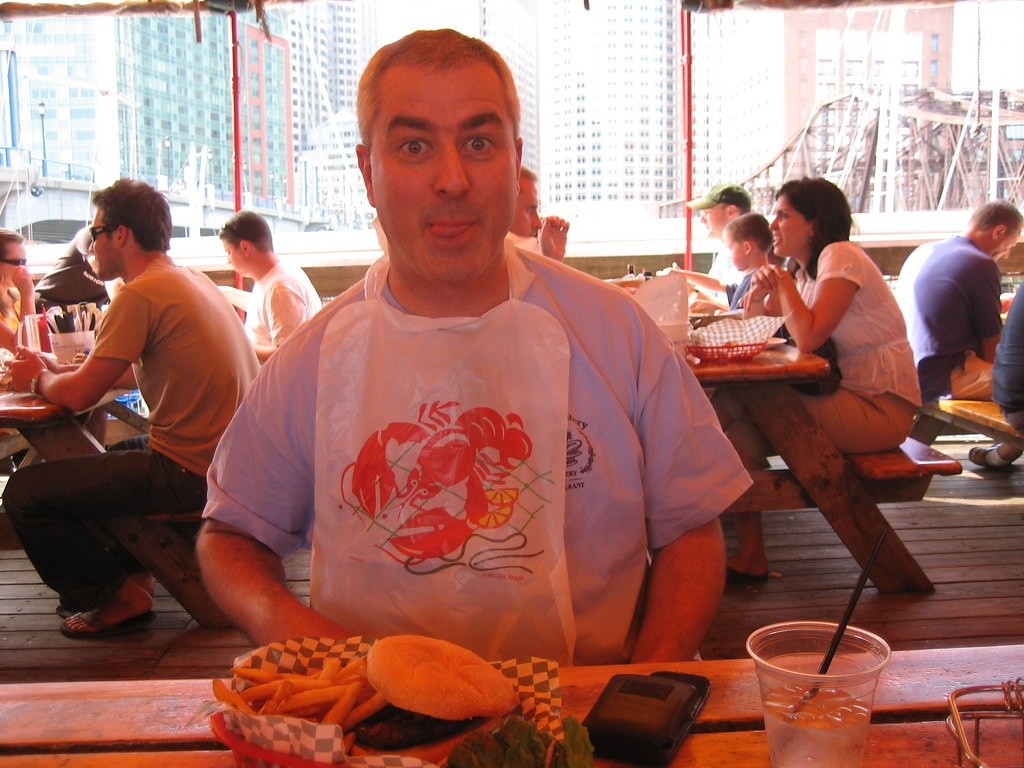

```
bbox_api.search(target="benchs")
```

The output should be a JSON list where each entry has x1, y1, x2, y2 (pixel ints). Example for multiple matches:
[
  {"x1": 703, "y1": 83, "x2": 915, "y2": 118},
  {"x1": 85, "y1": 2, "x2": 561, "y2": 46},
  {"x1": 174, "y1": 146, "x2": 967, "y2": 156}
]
[
  {"x1": 938, "y1": 400, "x2": 1022, "y2": 438},
  {"x1": 839, "y1": 438, "x2": 962, "y2": 595},
  {"x1": 144, "y1": 509, "x2": 234, "y2": 631}
]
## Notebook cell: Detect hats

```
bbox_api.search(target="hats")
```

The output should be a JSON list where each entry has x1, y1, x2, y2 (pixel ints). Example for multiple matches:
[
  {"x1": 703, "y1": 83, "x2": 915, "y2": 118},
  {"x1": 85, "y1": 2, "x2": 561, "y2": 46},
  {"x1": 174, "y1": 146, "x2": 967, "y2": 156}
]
[{"x1": 686, "y1": 183, "x2": 751, "y2": 213}]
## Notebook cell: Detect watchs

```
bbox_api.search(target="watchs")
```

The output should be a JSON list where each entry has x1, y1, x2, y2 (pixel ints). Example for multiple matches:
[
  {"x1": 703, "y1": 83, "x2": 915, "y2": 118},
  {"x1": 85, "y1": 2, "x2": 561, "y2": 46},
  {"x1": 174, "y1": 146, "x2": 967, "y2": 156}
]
[{"x1": 31, "y1": 370, "x2": 53, "y2": 398}]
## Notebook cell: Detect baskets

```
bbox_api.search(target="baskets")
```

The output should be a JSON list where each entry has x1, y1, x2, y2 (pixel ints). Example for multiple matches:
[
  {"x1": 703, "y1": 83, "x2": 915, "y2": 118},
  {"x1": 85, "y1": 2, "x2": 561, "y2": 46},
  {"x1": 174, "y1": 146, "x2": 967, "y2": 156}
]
[
  {"x1": 689, "y1": 342, "x2": 767, "y2": 365},
  {"x1": 212, "y1": 707, "x2": 501, "y2": 768}
]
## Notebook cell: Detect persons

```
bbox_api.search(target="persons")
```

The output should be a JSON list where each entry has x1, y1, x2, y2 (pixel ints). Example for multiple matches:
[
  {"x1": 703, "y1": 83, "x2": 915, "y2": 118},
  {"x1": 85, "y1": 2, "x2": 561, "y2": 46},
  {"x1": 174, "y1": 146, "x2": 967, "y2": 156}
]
[
  {"x1": 510, "y1": 166, "x2": 570, "y2": 263},
  {"x1": 684, "y1": 183, "x2": 752, "y2": 303},
  {"x1": 0, "y1": 228, "x2": 109, "y2": 448},
  {"x1": 2, "y1": 179, "x2": 260, "y2": 639},
  {"x1": 664, "y1": 213, "x2": 783, "y2": 339},
  {"x1": 895, "y1": 200, "x2": 1024, "y2": 467},
  {"x1": 34, "y1": 225, "x2": 119, "y2": 314},
  {"x1": 198, "y1": 30, "x2": 753, "y2": 664},
  {"x1": 992, "y1": 282, "x2": 1024, "y2": 432},
  {"x1": 709, "y1": 178, "x2": 921, "y2": 581},
  {"x1": 218, "y1": 210, "x2": 322, "y2": 367}
]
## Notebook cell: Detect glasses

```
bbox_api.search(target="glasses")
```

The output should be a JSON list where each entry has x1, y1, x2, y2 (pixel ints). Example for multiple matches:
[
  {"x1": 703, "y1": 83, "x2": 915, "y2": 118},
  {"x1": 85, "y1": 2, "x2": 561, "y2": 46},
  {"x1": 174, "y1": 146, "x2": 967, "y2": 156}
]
[
  {"x1": 0, "y1": 258, "x2": 27, "y2": 265},
  {"x1": 89, "y1": 227, "x2": 107, "y2": 240}
]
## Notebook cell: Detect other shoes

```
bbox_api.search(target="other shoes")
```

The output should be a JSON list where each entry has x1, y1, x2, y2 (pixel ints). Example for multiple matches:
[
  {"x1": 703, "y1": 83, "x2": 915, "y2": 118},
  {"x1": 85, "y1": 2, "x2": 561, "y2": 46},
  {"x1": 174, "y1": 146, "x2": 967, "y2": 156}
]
[{"x1": 969, "y1": 448, "x2": 995, "y2": 468}]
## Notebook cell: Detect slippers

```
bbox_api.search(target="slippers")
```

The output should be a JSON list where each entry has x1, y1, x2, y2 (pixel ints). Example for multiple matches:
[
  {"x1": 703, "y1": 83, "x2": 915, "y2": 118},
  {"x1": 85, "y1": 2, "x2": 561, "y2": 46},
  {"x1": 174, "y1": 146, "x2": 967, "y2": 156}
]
[
  {"x1": 727, "y1": 565, "x2": 768, "y2": 585},
  {"x1": 58, "y1": 605, "x2": 73, "y2": 618},
  {"x1": 60, "y1": 609, "x2": 157, "y2": 640}
]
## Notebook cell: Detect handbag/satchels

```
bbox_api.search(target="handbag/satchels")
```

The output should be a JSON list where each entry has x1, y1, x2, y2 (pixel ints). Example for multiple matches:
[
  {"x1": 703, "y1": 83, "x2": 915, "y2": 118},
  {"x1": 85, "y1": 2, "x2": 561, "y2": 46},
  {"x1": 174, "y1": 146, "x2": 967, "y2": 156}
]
[{"x1": 787, "y1": 334, "x2": 844, "y2": 396}]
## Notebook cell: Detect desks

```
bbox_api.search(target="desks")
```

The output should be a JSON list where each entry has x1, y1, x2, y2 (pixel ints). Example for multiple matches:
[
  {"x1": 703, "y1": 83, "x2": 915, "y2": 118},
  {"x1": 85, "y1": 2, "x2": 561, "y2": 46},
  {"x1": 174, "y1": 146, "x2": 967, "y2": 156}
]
[
  {"x1": 0, "y1": 644, "x2": 1024, "y2": 767},
  {"x1": 0, "y1": 387, "x2": 233, "y2": 632},
  {"x1": 690, "y1": 347, "x2": 936, "y2": 595}
]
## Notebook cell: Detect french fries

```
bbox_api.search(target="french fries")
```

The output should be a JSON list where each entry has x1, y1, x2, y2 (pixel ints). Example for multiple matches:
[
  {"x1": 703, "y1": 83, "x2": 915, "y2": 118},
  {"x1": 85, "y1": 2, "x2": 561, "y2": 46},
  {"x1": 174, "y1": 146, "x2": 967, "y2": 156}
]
[{"x1": 211, "y1": 655, "x2": 390, "y2": 758}]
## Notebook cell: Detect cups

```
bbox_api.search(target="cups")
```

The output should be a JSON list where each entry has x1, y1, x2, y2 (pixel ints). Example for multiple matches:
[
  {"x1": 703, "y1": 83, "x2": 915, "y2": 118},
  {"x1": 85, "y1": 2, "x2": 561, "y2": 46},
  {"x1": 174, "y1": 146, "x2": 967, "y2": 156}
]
[
  {"x1": 49, "y1": 330, "x2": 95, "y2": 365},
  {"x1": 745, "y1": 621, "x2": 891, "y2": 768}
]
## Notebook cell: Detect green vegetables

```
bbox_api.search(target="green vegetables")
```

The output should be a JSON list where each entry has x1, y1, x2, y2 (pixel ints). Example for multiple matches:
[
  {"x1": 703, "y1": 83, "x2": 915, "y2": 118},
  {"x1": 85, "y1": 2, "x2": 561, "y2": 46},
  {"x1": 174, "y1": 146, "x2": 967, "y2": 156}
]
[{"x1": 447, "y1": 712, "x2": 595, "y2": 768}]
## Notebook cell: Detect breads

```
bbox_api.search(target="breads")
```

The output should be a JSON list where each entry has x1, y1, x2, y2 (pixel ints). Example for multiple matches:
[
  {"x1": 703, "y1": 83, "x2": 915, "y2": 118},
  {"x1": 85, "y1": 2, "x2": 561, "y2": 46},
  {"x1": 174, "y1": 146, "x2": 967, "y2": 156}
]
[{"x1": 367, "y1": 635, "x2": 519, "y2": 720}]
